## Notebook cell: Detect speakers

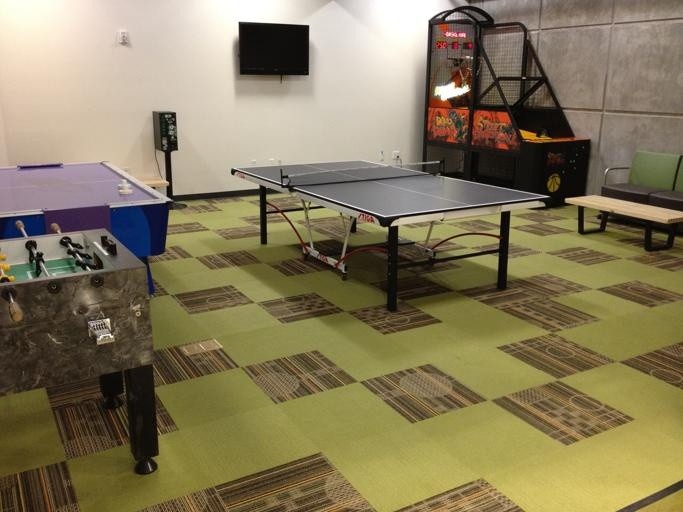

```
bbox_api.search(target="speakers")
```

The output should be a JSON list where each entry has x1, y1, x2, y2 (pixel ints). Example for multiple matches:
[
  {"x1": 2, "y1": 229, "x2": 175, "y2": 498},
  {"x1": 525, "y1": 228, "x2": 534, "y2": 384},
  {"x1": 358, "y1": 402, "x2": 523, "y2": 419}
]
[{"x1": 153, "y1": 111, "x2": 178, "y2": 152}]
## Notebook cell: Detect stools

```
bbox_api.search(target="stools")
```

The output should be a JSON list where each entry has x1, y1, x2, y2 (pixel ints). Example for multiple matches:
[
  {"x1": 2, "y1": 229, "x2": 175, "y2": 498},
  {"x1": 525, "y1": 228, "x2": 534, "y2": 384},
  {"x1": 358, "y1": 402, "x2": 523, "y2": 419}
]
[{"x1": 134, "y1": 177, "x2": 170, "y2": 190}]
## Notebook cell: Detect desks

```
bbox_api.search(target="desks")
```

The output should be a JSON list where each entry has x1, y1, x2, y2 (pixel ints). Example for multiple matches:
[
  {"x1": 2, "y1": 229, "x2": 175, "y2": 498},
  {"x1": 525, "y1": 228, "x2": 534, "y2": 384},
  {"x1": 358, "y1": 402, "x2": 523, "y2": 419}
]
[
  {"x1": 230, "y1": 159, "x2": 552, "y2": 315},
  {"x1": 564, "y1": 194, "x2": 683, "y2": 250},
  {"x1": 0, "y1": 159, "x2": 173, "y2": 296}
]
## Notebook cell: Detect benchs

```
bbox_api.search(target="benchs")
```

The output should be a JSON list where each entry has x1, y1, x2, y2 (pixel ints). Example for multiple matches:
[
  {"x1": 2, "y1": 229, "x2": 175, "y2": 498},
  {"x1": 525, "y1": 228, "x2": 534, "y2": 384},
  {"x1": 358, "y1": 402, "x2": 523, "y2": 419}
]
[{"x1": 600, "y1": 150, "x2": 683, "y2": 210}]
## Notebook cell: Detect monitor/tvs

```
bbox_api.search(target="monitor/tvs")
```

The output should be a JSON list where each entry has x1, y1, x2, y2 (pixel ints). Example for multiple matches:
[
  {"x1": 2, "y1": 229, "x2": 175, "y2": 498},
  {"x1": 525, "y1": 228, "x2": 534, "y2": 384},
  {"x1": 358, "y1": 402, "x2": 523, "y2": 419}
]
[{"x1": 239, "y1": 22, "x2": 309, "y2": 75}]
